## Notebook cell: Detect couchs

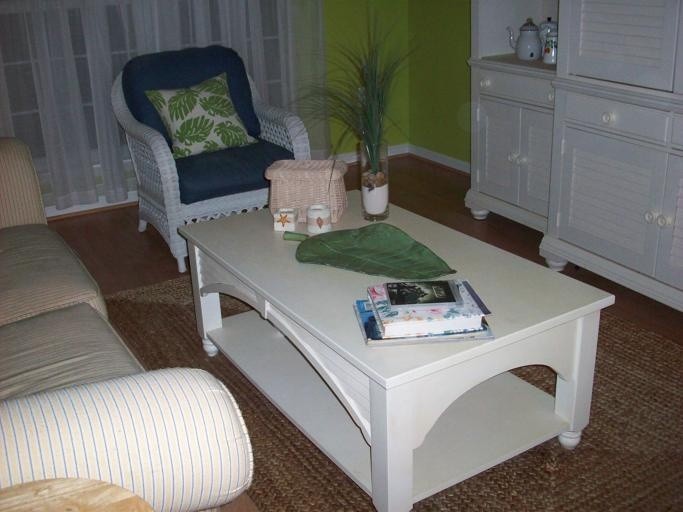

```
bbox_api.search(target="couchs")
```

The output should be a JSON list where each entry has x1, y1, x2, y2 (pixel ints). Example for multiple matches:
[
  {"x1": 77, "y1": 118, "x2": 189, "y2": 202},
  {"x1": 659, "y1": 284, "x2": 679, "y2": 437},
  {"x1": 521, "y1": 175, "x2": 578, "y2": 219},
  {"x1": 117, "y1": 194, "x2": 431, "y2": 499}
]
[{"x1": 0, "y1": 139, "x2": 253, "y2": 512}]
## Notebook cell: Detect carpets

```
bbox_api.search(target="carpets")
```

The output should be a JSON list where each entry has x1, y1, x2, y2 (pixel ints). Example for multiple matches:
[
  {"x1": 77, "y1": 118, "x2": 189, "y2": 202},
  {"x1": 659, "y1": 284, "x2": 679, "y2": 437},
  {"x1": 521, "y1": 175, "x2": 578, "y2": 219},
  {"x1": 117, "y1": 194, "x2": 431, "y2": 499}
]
[{"x1": 99, "y1": 269, "x2": 683, "y2": 512}]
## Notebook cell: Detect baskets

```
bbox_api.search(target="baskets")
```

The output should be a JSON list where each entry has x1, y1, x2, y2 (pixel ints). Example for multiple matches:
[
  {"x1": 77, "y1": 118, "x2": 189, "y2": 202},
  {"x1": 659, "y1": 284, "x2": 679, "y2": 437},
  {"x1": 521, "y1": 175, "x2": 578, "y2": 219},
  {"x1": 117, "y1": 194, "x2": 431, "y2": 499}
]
[{"x1": 265, "y1": 159, "x2": 349, "y2": 224}]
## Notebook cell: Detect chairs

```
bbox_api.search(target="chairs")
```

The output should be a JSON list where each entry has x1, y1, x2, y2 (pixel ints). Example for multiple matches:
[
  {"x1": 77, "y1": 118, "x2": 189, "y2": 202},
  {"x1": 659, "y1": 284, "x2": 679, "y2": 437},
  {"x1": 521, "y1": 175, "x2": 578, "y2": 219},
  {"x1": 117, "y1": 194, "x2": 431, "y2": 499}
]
[{"x1": 111, "y1": 44, "x2": 309, "y2": 269}]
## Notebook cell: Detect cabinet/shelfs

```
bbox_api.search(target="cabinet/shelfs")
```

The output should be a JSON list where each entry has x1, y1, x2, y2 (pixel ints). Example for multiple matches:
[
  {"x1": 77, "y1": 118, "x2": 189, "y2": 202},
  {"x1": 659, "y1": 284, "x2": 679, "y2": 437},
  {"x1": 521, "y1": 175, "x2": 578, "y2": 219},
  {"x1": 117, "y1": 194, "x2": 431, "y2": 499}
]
[{"x1": 467, "y1": 0, "x2": 683, "y2": 313}]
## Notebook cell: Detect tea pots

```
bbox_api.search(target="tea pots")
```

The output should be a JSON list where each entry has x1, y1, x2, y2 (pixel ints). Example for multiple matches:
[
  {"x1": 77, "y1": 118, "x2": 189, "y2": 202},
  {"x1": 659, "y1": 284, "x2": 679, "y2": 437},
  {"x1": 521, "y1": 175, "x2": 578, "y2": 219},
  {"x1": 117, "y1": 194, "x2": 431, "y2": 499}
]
[{"x1": 506, "y1": 18, "x2": 552, "y2": 63}]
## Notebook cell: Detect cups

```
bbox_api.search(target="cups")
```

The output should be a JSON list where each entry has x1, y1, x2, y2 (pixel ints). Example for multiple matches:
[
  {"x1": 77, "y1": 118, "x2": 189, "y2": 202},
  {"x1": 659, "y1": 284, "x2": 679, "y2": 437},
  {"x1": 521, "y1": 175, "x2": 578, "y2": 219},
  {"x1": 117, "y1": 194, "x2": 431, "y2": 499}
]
[{"x1": 307, "y1": 204, "x2": 332, "y2": 234}]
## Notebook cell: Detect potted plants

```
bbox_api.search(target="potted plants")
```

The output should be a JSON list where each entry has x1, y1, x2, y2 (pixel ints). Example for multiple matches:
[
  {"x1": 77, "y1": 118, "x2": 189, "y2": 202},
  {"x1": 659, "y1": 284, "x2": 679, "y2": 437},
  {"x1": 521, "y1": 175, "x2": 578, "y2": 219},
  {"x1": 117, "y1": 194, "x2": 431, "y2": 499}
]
[{"x1": 280, "y1": 8, "x2": 419, "y2": 220}]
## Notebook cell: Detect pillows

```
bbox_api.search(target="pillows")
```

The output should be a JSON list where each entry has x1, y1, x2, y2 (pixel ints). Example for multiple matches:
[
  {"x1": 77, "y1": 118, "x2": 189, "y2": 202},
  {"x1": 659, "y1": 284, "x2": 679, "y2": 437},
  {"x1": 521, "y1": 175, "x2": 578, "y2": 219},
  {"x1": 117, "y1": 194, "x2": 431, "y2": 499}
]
[{"x1": 144, "y1": 71, "x2": 259, "y2": 160}]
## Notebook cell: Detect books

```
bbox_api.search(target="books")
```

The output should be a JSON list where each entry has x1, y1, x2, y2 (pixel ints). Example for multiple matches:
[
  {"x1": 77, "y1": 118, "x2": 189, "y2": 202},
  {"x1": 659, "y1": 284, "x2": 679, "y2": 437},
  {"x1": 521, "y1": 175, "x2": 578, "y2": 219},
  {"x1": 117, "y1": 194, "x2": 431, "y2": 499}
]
[
  {"x1": 384, "y1": 279, "x2": 462, "y2": 310},
  {"x1": 367, "y1": 278, "x2": 491, "y2": 337},
  {"x1": 351, "y1": 298, "x2": 492, "y2": 345}
]
